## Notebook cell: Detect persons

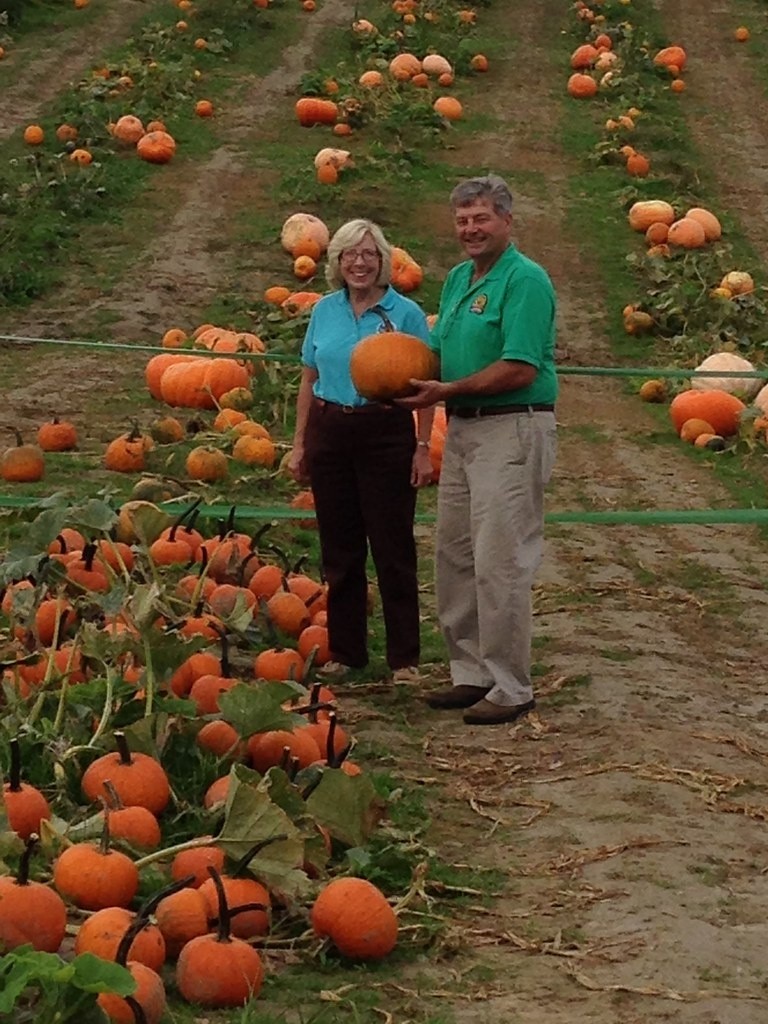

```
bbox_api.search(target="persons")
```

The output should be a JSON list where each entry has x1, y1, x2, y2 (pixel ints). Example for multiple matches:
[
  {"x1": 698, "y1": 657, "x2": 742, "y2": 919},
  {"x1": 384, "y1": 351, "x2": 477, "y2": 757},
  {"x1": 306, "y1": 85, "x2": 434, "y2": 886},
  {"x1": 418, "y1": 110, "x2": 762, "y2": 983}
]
[
  {"x1": 276, "y1": 218, "x2": 433, "y2": 687},
  {"x1": 392, "y1": 175, "x2": 557, "y2": 723}
]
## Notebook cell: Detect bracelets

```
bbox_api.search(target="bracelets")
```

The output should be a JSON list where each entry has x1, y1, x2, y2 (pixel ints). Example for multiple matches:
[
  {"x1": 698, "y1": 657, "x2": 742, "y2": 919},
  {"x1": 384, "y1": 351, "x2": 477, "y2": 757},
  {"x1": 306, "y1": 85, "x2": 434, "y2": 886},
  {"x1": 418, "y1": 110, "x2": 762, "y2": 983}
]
[{"x1": 418, "y1": 441, "x2": 430, "y2": 448}]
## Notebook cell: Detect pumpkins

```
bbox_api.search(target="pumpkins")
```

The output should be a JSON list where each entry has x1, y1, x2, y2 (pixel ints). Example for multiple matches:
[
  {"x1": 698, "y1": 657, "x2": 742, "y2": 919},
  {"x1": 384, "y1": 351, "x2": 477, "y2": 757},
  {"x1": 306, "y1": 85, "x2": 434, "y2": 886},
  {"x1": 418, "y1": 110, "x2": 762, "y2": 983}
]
[{"x1": 0, "y1": 0, "x2": 767, "y2": 1023}]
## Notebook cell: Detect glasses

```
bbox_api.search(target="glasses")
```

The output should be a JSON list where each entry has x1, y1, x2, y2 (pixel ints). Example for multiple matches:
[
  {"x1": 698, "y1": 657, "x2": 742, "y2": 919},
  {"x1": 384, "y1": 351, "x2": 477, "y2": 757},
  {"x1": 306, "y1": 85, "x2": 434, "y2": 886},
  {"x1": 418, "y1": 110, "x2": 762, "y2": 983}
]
[{"x1": 341, "y1": 249, "x2": 379, "y2": 262}]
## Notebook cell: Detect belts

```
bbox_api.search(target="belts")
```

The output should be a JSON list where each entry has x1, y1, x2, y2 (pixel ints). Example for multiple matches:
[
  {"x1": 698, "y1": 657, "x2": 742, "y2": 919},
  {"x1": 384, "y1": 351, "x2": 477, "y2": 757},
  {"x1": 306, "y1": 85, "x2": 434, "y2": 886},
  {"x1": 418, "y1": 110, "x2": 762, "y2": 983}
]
[
  {"x1": 448, "y1": 404, "x2": 554, "y2": 418},
  {"x1": 314, "y1": 398, "x2": 398, "y2": 414}
]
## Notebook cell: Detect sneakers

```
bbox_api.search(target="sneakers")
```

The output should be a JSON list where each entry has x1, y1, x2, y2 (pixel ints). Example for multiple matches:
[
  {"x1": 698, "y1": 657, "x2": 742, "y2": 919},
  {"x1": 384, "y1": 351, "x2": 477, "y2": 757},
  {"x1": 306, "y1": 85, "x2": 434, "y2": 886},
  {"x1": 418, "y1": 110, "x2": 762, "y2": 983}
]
[
  {"x1": 393, "y1": 665, "x2": 421, "y2": 684},
  {"x1": 429, "y1": 685, "x2": 491, "y2": 709},
  {"x1": 463, "y1": 697, "x2": 536, "y2": 724},
  {"x1": 315, "y1": 660, "x2": 352, "y2": 679}
]
